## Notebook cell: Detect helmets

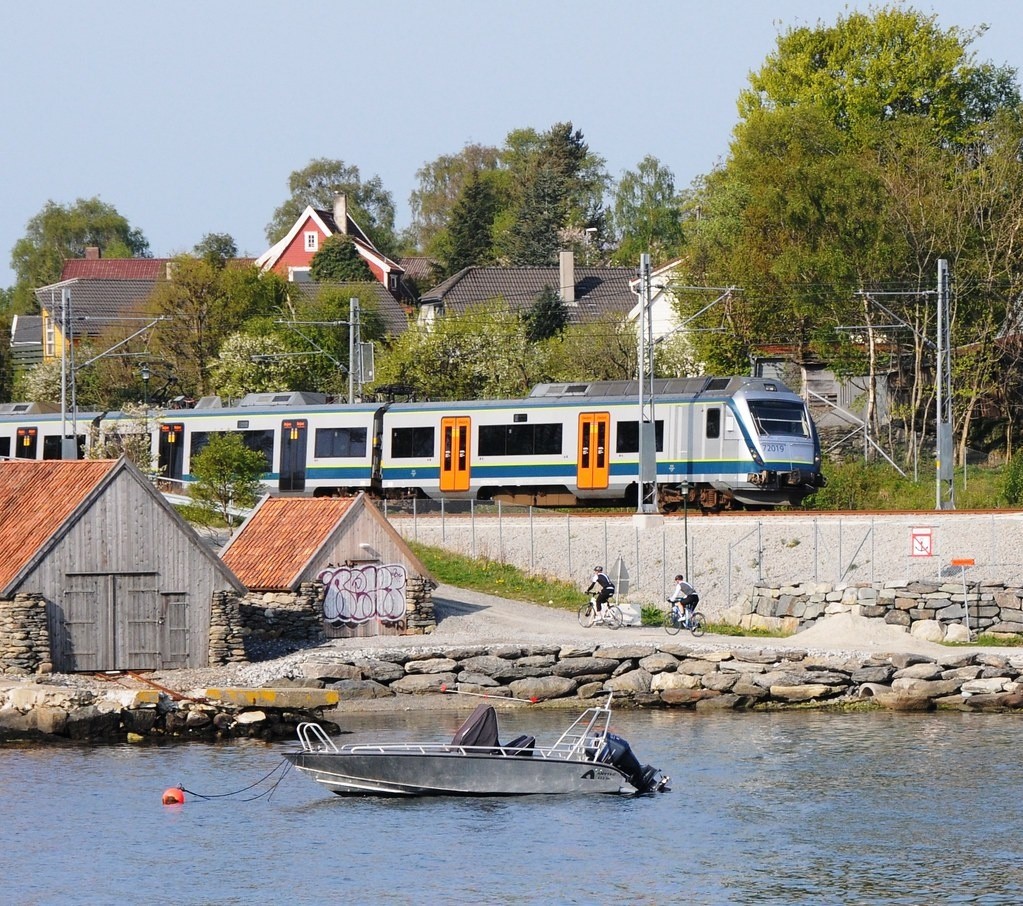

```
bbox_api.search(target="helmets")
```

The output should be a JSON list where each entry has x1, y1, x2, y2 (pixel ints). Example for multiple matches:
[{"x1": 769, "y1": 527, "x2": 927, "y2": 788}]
[{"x1": 594, "y1": 566, "x2": 603, "y2": 571}]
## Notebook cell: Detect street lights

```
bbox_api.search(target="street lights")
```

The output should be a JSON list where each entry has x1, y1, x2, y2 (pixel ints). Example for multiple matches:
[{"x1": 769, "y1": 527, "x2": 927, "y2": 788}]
[{"x1": 142, "y1": 368, "x2": 150, "y2": 404}]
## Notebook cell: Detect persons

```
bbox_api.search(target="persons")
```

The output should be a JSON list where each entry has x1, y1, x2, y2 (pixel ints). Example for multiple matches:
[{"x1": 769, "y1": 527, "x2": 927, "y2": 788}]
[
  {"x1": 669, "y1": 575, "x2": 699, "y2": 632},
  {"x1": 586, "y1": 566, "x2": 614, "y2": 621}
]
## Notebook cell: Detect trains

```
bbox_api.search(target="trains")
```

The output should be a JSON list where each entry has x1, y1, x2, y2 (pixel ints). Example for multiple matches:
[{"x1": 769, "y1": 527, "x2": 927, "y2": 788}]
[{"x1": 0, "y1": 376, "x2": 823, "y2": 516}]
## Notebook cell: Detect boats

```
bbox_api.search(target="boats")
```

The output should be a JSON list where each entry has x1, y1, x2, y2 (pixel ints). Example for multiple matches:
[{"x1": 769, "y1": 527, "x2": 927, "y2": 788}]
[{"x1": 281, "y1": 692, "x2": 672, "y2": 797}]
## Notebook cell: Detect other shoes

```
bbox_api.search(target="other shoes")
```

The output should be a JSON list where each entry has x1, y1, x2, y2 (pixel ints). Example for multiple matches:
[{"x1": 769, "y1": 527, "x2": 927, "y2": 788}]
[
  {"x1": 678, "y1": 617, "x2": 686, "y2": 622},
  {"x1": 593, "y1": 616, "x2": 602, "y2": 622}
]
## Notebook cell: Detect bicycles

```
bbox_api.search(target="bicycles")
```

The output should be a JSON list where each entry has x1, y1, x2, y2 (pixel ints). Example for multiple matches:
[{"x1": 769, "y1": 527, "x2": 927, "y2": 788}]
[
  {"x1": 578, "y1": 590, "x2": 624, "y2": 630},
  {"x1": 663, "y1": 597, "x2": 706, "y2": 636}
]
